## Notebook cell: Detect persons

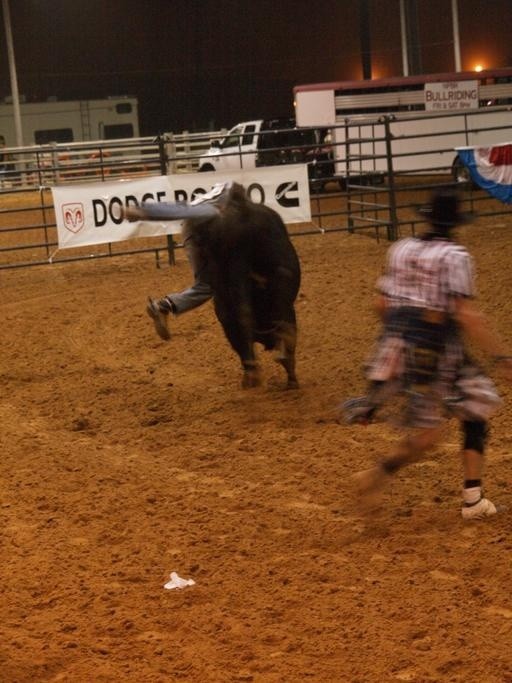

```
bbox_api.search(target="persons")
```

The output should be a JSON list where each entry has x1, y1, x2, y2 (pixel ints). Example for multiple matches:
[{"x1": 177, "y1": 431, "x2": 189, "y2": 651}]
[
  {"x1": 338, "y1": 187, "x2": 497, "y2": 523},
  {"x1": 120, "y1": 177, "x2": 250, "y2": 340}
]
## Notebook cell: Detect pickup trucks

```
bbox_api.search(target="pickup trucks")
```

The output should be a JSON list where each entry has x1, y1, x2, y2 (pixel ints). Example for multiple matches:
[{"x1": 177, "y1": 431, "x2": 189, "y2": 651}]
[{"x1": 199, "y1": 114, "x2": 336, "y2": 193}]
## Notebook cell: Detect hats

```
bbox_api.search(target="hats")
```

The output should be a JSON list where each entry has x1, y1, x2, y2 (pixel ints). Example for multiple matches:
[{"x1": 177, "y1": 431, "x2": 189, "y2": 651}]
[{"x1": 408, "y1": 189, "x2": 475, "y2": 228}]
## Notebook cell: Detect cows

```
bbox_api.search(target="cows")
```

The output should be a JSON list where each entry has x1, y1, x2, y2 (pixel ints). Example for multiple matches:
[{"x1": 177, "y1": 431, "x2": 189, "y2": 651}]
[{"x1": 193, "y1": 183, "x2": 302, "y2": 393}]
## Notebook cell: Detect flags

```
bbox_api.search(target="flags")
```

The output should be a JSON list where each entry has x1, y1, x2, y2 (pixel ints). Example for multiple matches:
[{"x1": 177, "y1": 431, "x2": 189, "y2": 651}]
[{"x1": 458, "y1": 144, "x2": 511, "y2": 205}]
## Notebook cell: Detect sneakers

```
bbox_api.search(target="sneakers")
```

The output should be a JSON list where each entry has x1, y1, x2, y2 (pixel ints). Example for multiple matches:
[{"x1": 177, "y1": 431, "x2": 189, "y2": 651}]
[
  {"x1": 144, "y1": 296, "x2": 173, "y2": 343},
  {"x1": 458, "y1": 483, "x2": 497, "y2": 523}
]
